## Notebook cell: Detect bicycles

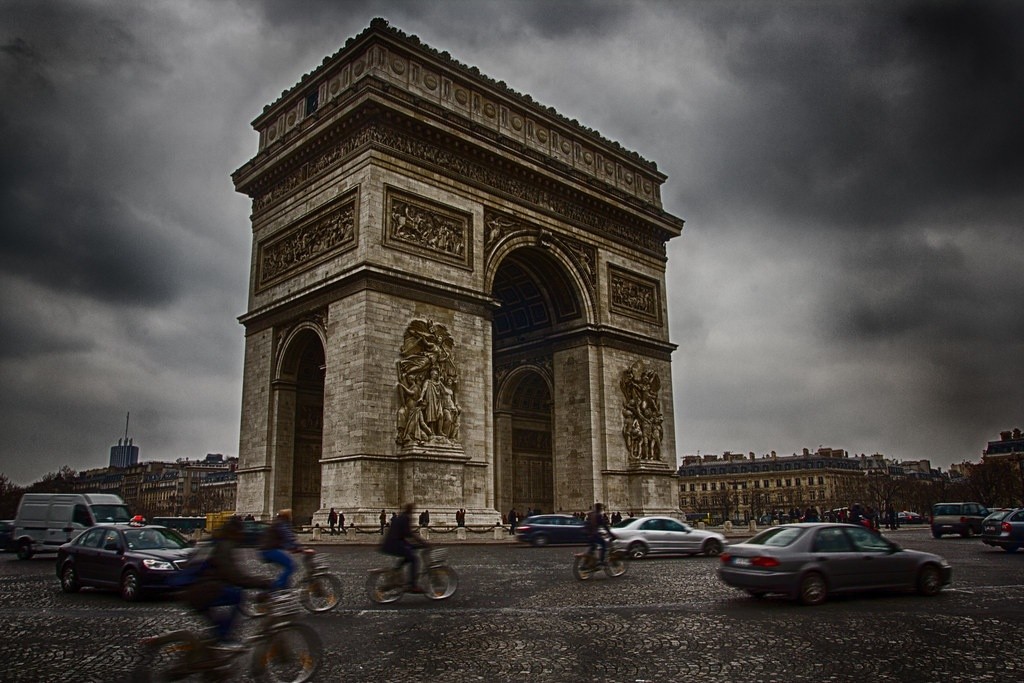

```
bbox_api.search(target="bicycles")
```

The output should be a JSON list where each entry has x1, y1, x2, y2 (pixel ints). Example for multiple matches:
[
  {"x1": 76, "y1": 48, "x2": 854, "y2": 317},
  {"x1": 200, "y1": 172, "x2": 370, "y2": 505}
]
[
  {"x1": 238, "y1": 547, "x2": 342, "y2": 618},
  {"x1": 137, "y1": 590, "x2": 322, "y2": 683},
  {"x1": 573, "y1": 533, "x2": 628, "y2": 581},
  {"x1": 366, "y1": 543, "x2": 459, "y2": 604}
]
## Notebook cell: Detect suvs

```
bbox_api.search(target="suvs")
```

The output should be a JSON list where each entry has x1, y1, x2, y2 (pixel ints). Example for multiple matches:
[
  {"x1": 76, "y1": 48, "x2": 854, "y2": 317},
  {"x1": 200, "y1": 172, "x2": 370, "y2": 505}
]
[
  {"x1": 982, "y1": 508, "x2": 1024, "y2": 551},
  {"x1": 930, "y1": 503, "x2": 990, "y2": 538}
]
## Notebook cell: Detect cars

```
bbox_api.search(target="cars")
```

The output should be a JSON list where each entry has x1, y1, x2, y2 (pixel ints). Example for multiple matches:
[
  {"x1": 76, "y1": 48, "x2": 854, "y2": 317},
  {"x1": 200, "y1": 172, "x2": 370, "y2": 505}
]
[
  {"x1": 599, "y1": 516, "x2": 728, "y2": 560},
  {"x1": 897, "y1": 512, "x2": 924, "y2": 523},
  {"x1": 716, "y1": 522, "x2": 953, "y2": 604},
  {"x1": 55, "y1": 525, "x2": 202, "y2": 599},
  {"x1": 514, "y1": 514, "x2": 608, "y2": 546},
  {"x1": 0, "y1": 520, "x2": 15, "y2": 549}
]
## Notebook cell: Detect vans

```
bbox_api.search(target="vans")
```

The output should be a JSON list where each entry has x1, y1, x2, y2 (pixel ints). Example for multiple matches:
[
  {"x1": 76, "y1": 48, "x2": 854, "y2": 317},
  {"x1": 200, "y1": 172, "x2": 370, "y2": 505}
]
[{"x1": 13, "y1": 493, "x2": 133, "y2": 560}]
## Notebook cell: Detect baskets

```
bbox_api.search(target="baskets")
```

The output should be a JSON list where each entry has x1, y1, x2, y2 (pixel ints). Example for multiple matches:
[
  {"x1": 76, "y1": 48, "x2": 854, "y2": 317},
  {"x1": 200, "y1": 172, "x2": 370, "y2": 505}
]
[
  {"x1": 261, "y1": 587, "x2": 303, "y2": 618},
  {"x1": 302, "y1": 553, "x2": 330, "y2": 571},
  {"x1": 422, "y1": 546, "x2": 448, "y2": 565}
]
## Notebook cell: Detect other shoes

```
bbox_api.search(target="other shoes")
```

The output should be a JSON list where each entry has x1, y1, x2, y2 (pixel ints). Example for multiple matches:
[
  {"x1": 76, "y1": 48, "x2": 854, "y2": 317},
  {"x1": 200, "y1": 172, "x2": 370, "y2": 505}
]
[{"x1": 410, "y1": 587, "x2": 425, "y2": 594}]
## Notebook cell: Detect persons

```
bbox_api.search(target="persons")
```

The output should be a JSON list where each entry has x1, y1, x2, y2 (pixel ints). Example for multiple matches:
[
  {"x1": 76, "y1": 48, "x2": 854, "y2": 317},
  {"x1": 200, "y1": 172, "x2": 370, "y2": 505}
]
[
  {"x1": 379, "y1": 504, "x2": 428, "y2": 594},
  {"x1": 567, "y1": 244, "x2": 593, "y2": 285},
  {"x1": 627, "y1": 511, "x2": 635, "y2": 517},
  {"x1": 771, "y1": 508, "x2": 801, "y2": 524},
  {"x1": 573, "y1": 512, "x2": 586, "y2": 520},
  {"x1": 244, "y1": 514, "x2": 255, "y2": 520},
  {"x1": 888, "y1": 503, "x2": 897, "y2": 530},
  {"x1": 398, "y1": 329, "x2": 462, "y2": 437},
  {"x1": 503, "y1": 514, "x2": 508, "y2": 524},
  {"x1": 829, "y1": 508, "x2": 844, "y2": 522},
  {"x1": 258, "y1": 508, "x2": 307, "y2": 593},
  {"x1": 623, "y1": 369, "x2": 666, "y2": 461},
  {"x1": 379, "y1": 509, "x2": 429, "y2": 535},
  {"x1": 485, "y1": 216, "x2": 515, "y2": 251},
  {"x1": 182, "y1": 515, "x2": 278, "y2": 670},
  {"x1": 603, "y1": 512, "x2": 622, "y2": 522},
  {"x1": 508, "y1": 507, "x2": 516, "y2": 535},
  {"x1": 849, "y1": 503, "x2": 873, "y2": 525},
  {"x1": 516, "y1": 512, "x2": 525, "y2": 522},
  {"x1": 581, "y1": 503, "x2": 620, "y2": 570},
  {"x1": 456, "y1": 508, "x2": 465, "y2": 526},
  {"x1": 327, "y1": 508, "x2": 347, "y2": 535},
  {"x1": 527, "y1": 506, "x2": 533, "y2": 515},
  {"x1": 231, "y1": 513, "x2": 243, "y2": 521},
  {"x1": 805, "y1": 505, "x2": 818, "y2": 522}
]
